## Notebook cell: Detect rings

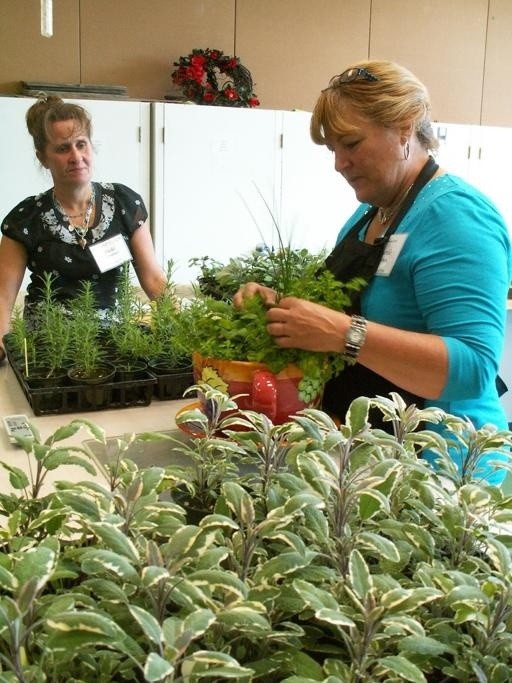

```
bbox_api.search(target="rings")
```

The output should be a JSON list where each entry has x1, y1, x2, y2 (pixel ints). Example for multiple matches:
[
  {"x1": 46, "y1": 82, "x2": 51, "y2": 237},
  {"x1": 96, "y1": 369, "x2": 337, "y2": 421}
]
[{"x1": 280, "y1": 321, "x2": 289, "y2": 336}]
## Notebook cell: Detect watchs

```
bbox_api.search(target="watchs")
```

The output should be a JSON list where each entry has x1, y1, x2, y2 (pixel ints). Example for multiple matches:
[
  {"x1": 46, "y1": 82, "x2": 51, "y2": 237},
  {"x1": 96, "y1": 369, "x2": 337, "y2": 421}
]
[{"x1": 344, "y1": 311, "x2": 367, "y2": 365}]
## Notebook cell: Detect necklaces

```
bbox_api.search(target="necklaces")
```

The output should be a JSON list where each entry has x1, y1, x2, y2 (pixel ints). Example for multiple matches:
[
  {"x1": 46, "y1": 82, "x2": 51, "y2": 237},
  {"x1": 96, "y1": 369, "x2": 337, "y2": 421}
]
[
  {"x1": 53, "y1": 188, "x2": 88, "y2": 218},
  {"x1": 55, "y1": 183, "x2": 95, "y2": 250},
  {"x1": 378, "y1": 182, "x2": 412, "y2": 223}
]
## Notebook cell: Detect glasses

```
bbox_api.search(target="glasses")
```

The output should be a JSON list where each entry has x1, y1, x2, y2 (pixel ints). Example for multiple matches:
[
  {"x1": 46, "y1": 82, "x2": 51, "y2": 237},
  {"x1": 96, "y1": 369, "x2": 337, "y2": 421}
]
[{"x1": 327, "y1": 68, "x2": 379, "y2": 90}]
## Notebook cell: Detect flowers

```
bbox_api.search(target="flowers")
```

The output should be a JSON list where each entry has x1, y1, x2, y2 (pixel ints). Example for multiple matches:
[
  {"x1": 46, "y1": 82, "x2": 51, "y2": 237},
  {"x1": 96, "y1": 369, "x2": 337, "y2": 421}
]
[{"x1": 172, "y1": 46, "x2": 260, "y2": 108}]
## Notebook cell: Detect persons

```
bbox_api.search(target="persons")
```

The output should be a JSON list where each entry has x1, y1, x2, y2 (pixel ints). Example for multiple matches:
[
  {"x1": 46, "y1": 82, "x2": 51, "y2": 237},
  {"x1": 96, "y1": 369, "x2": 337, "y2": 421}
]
[
  {"x1": 232, "y1": 59, "x2": 510, "y2": 486},
  {"x1": 1, "y1": 91, "x2": 182, "y2": 365}
]
